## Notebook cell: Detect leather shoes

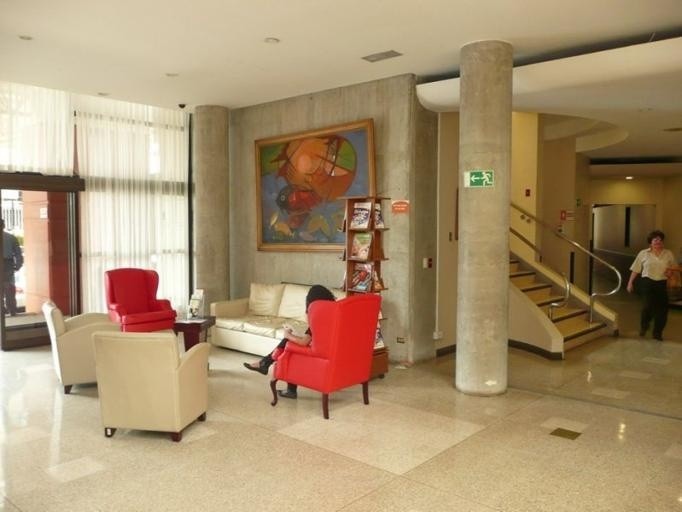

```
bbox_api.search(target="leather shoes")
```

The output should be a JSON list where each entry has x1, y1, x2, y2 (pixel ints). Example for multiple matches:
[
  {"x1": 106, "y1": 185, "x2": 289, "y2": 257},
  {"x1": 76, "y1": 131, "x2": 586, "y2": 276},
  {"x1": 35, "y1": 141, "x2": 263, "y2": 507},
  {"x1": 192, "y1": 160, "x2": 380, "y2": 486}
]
[
  {"x1": 242, "y1": 361, "x2": 268, "y2": 376},
  {"x1": 639, "y1": 323, "x2": 650, "y2": 336},
  {"x1": 653, "y1": 334, "x2": 663, "y2": 340},
  {"x1": 277, "y1": 389, "x2": 297, "y2": 399}
]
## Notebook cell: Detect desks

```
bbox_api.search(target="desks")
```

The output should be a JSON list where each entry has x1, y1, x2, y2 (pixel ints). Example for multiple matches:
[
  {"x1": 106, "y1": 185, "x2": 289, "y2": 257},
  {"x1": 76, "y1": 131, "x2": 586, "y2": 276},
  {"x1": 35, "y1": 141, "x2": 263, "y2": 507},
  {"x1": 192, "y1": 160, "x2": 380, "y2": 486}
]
[{"x1": 173, "y1": 315, "x2": 216, "y2": 352}]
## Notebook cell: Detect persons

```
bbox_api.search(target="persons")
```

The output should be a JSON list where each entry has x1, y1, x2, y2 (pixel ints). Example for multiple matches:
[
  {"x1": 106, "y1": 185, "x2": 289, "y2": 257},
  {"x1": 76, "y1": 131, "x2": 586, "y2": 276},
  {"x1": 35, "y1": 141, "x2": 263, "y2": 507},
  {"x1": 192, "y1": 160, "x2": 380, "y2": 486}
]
[
  {"x1": 627, "y1": 230, "x2": 676, "y2": 342},
  {"x1": 667, "y1": 260, "x2": 682, "y2": 289},
  {"x1": 243, "y1": 285, "x2": 335, "y2": 400},
  {"x1": 0, "y1": 219, "x2": 24, "y2": 317}
]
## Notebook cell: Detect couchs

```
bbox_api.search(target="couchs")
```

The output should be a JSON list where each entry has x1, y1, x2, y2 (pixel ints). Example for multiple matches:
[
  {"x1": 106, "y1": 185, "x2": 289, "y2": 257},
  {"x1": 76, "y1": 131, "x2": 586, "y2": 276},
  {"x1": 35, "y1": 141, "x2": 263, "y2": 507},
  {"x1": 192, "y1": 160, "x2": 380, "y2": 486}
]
[
  {"x1": 92, "y1": 330, "x2": 212, "y2": 442},
  {"x1": 269, "y1": 290, "x2": 384, "y2": 422},
  {"x1": 210, "y1": 281, "x2": 347, "y2": 358},
  {"x1": 104, "y1": 267, "x2": 177, "y2": 333},
  {"x1": 42, "y1": 298, "x2": 122, "y2": 394}
]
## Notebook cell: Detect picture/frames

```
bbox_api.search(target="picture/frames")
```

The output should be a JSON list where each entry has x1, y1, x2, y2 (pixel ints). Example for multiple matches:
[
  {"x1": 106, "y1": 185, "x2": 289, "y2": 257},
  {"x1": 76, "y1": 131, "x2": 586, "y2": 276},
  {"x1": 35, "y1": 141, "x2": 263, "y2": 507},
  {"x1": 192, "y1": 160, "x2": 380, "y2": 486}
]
[{"x1": 253, "y1": 117, "x2": 377, "y2": 252}]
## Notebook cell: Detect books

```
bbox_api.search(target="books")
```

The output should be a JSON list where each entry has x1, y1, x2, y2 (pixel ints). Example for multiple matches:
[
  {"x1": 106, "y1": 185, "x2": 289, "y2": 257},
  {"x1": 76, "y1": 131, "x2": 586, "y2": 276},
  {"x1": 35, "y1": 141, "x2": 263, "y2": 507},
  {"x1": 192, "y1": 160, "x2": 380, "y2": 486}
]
[
  {"x1": 187, "y1": 286, "x2": 204, "y2": 317},
  {"x1": 350, "y1": 203, "x2": 387, "y2": 349}
]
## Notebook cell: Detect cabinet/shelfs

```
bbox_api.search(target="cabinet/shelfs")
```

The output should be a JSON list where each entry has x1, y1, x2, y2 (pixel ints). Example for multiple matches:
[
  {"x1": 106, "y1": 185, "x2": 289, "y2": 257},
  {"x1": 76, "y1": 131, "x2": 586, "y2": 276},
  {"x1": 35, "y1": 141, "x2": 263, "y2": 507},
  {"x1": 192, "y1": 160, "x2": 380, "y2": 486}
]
[{"x1": 333, "y1": 195, "x2": 391, "y2": 389}]
